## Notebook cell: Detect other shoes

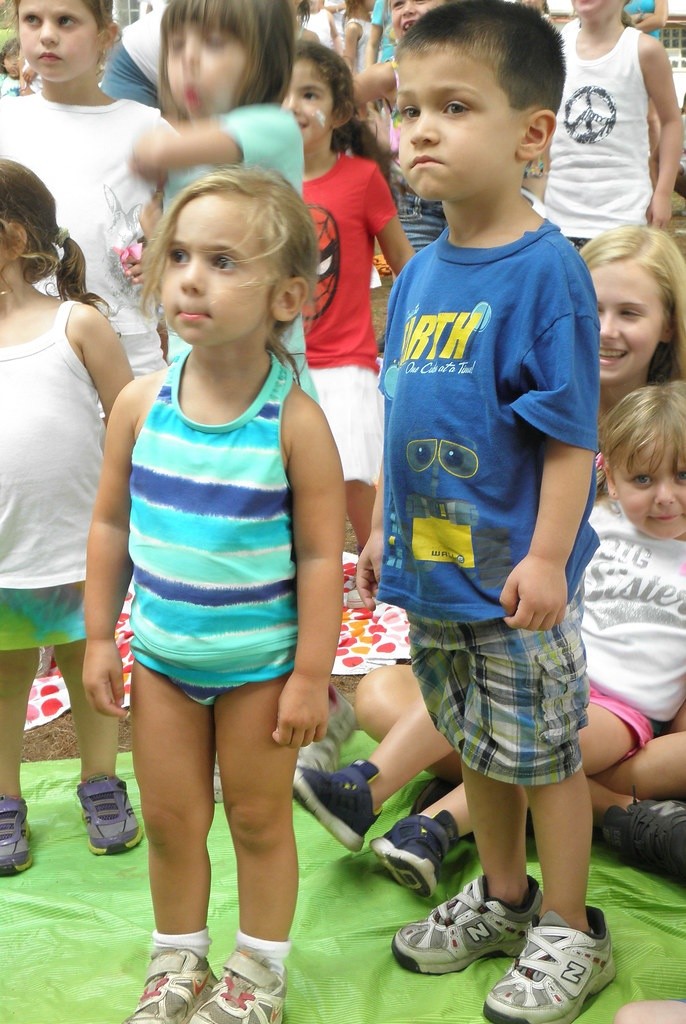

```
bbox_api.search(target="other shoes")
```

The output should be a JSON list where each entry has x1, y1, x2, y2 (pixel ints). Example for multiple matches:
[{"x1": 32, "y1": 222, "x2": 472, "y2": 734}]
[
  {"x1": 375, "y1": 333, "x2": 385, "y2": 353},
  {"x1": 213, "y1": 758, "x2": 225, "y2": 804},
  {"x1": 295, "y1": 683, "x2": 355, "y2": 775},
  {"x1": 347, "y1": 545, "x2": 366, "y2": 609}
]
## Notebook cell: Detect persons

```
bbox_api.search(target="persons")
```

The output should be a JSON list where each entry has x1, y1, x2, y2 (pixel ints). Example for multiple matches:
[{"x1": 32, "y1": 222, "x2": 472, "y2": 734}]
[
  {"x1": 81, "y1": 165, "x2": 346, "y2": 1024},
  {"x1": 356, "y1": 1, "x2": 617, "y2": 1024},
  {"x1": 0, "y1": 0, "x2": 686, "y2": 893}
]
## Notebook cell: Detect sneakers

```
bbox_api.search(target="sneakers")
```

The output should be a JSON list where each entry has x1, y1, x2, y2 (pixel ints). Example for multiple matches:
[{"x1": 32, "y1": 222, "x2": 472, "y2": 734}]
[
  {"x1": 602, "y1": 784, "x2": 686, "y2": 883},
  {"x1": 483, "y1": 905, "x2": 616, "y2": 1024},
  {"x1": 184, "y1": 949, "x2": 288, "y2": 1024},
  {"x1": 76, "y1": 774, "x2": 143, "y2": 855},
  {"x1": 390, "y1": 874, "x2": 543, "y2": 975},
  {"x1": 0, "y1": 793, "x2": 32, "y2": 876},
  {"x1": 292, "y1": 758, "x2": 384, "y2": 852},
  {"x1": 119, "y1": 949, "x2": 218, "y2": 1024},
  {"x1": 409, "y1": 776, "x2": 475, "y2": 844},
  {"x1": 369, "y1": 809, "x2": 459, "y2": 898}
]
